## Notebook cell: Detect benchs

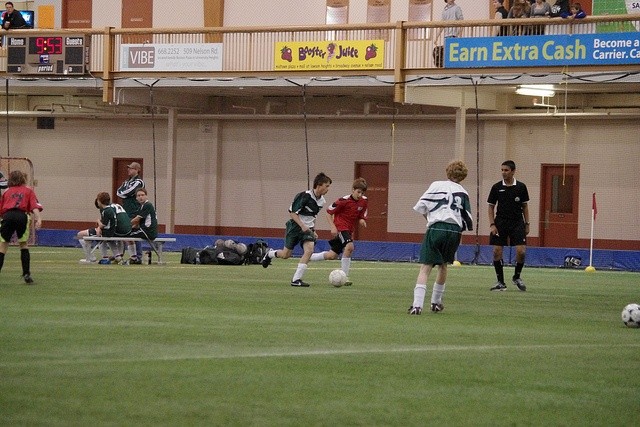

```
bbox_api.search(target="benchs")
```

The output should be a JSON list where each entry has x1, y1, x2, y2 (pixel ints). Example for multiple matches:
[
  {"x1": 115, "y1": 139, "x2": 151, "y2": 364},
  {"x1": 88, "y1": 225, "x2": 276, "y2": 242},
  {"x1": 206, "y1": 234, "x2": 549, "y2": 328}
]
[{"x1": 83, "y1": 236, "x2": 177, "y2": 265}]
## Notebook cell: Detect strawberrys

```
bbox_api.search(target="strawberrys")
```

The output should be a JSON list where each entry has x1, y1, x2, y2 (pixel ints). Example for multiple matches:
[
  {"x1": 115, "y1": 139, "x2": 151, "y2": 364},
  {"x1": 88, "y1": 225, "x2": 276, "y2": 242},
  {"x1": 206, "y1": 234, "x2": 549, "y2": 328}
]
[
  {"x1": 365, "y1": 43, "x2": 378, "y2": 61},
  {"x1": 280, "y1": 45, "x2": 293, "y2": 62},
  {"x1": 326, "y1": 43, "x2": 335, "y2": 54}
]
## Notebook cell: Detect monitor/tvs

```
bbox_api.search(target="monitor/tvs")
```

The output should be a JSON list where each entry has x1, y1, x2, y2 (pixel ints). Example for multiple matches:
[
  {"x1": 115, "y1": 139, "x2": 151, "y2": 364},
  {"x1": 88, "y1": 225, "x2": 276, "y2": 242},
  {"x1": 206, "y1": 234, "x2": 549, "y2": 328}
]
[{"x1": 18, "y1": 10, "x2": 34, "y2": 27}]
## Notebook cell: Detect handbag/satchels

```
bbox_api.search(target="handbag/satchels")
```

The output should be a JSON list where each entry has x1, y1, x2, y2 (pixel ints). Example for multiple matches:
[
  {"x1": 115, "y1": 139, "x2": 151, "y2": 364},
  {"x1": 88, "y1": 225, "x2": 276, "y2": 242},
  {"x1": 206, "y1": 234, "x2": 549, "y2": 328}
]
[{"x1": 181, "y1": 241, "x2": 268, "y2": 266}]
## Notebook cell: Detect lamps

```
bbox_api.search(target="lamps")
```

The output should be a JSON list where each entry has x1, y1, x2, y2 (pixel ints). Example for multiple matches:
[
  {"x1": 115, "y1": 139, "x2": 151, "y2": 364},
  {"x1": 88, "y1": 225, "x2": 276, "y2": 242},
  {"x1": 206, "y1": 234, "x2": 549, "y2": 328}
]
[{"x1": 514, "y1": 87, "x2": 556, "y2": 98}]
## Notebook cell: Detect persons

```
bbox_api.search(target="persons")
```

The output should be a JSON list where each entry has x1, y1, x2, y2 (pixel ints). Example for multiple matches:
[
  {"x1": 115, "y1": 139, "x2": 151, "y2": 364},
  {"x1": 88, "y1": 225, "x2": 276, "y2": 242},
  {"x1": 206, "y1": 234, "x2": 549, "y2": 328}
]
[
  {"x1": 110, "y1": 188, "x2": 158, "y2": 265},
  {"x1": 530, "y1": 0, "x2": 552, "y2": 35},
  {"x1": 525, "y1": 222, "x2": 530, "y2": 225},
  {"x1": 116, "y1": 162, "x2": 145, "y2": 264},
  {"x1": 506, "y1": 0, "x2": 533, "y2": 36},
  {"x1": 408, "y1": 160, "x2": 473, "y2": 314},
  {"x1": 309, "y1": 177, "x2": 368, "y2": 286},
  {"x1": 492, "y1": 0, "x2": 509, "y2": 36},
  {"x1": 433, "y1": 0, "x2": 464, "y2": 47},
  {"x1": 2, "y1": 2, "x2": 30, "y2": 72},
  {"x1": 0, "y1": 172, "x2": 5, "y2": 197},
  {"x1": 486, "y1": 160, "x2": 531, "y2": 292},
  {"x1": 561, "y1": 2, "x2": 586, "y2": 21},
  {"x1": 263, "y1": 172, "x2": 333, "y2": 287},
  {"x1": 545, "y1": 0, "x2": 570, "y2": 18},
  {"x1": 0, "y1": 170, "x2": 44, "y2": 283},
  {"x1": 77, "y1": 192, "x2": 118, "y2": 263},
  {"x1": 490, "y1": 223, "x2": 494, "y2": 227},
  {"x1": 95, "y1": 198, "x2": 132, "y2": 265}
]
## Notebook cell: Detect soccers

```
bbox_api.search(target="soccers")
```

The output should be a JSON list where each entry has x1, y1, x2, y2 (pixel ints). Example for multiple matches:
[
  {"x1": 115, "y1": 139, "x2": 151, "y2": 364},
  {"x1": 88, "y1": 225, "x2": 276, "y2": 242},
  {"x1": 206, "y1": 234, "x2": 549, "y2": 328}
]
[
  {"x1": 225, "y1": 238, "x2": 237, "y2": 252},
  {"x1": 329, "y1": 270, "x2": 347, "y2": 288},
  {"x1": 236, "y1": 242, "x2": 248, "y2": 257},
  {"x1": 214, "y1": 238, "x2": 225, "y2": 251},
  {"x1": 622, "y1": 303, "x2": 640, "y2": 328}
]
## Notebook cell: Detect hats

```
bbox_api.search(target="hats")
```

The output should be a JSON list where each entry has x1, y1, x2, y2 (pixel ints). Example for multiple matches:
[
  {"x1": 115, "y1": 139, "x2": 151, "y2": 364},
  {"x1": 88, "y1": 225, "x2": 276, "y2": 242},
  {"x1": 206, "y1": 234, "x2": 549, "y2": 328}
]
[{"x1": 126, "y1": 161, "x2": 141, "y2": 169}]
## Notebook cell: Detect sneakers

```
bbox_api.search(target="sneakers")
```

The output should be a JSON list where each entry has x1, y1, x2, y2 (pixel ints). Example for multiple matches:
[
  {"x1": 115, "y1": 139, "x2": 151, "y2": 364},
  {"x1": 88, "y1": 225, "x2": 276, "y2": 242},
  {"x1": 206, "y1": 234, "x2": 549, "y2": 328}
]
[
  {"x1": 290, "y1": 279, "x2": 310, "y2": 287},
  {"x1": 24, "y1": 274, "x2": 33, "y2": 284},
  {"x1": 408, "y1": 305, "x2": 422, "y2": 316},
  {"x1": 109, "y1": 255, "x2": 115, "y2": 261},
  {"x1": 79, "y1": 258, "x2": 97, "y2": 264},
  {"x1": 130, "y1": 254, "x2": 141, "y2": 264},
  {"x1": 511, "y1": 275, "x2": 527, "y2": 292},
  {"x1": 111, "y1": 254, "x2": 123, "y2": 264},
  {"x1": 489, "y1": 281, "x2": 508, "y2": 292},
  {"x1": 430, "y1": 303, "x2": 444, "y2": 313},
  {"x1": 262, "y1": 247, "x2": 273, "y2": 268}
]
